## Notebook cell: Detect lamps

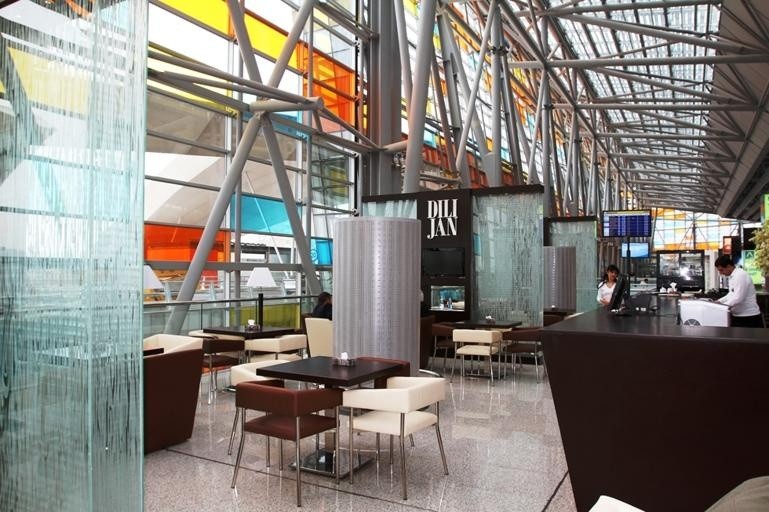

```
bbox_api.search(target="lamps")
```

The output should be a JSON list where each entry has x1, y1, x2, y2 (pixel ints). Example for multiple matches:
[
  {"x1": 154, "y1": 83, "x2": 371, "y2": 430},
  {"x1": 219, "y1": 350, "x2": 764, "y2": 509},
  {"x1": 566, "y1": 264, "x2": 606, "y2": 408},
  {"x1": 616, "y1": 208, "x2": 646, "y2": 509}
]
[
  {"x1": 248, "y1": 266, "x2": 275, "y2": 324},
  {"x1": 122, "y1": 263, "x2": 165, "y2": 346}
]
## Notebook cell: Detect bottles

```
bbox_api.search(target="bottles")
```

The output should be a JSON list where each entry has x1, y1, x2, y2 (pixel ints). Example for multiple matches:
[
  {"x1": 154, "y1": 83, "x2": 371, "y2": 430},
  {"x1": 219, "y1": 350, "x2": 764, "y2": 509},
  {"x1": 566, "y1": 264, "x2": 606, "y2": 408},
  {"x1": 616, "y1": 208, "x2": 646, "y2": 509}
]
[
  {"x1": 440, "y1": 296, "x2": 444, "y2": 308},
  {"x1": 448, "y1": 297, "x2": 452, "y2": 309}
]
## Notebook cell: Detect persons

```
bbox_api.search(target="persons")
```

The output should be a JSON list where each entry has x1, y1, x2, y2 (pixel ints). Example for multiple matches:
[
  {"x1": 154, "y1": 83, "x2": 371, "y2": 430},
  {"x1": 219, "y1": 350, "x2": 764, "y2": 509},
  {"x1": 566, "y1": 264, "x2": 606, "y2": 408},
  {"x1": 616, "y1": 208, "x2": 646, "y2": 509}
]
[
  {"x1": 597, "y1": 264, "x2": 625, "y2": 307},
  {"x1": 640, "y1": 279, "x2": 648, "y2": 284},
  {"x1": 312, "y1": 291, "x2": 332, "y2": 319},
  {"x1": 714, "y1": 255, "x2": 763, "y2": 326}
]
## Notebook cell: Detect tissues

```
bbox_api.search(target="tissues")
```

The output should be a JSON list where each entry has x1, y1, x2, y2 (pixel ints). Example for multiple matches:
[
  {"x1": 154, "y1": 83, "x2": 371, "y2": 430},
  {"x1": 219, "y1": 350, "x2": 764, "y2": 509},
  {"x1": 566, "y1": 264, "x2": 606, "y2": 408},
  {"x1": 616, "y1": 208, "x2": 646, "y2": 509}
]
[
  {"x1": 486, "y1": 316, "x2": 495, "y2": 324},
  {"x1": 669, "y1": 282, "x2": 681, "y2": 298},
  {"x1": 333, "y1": 352, "x2": 356, "y2": 367},
  {"x1": 551, "y1": 304, "x2": 559, "y2": 311},
  {"x1": 245, "y1": 319, "x2": 261, "y2": 330}
]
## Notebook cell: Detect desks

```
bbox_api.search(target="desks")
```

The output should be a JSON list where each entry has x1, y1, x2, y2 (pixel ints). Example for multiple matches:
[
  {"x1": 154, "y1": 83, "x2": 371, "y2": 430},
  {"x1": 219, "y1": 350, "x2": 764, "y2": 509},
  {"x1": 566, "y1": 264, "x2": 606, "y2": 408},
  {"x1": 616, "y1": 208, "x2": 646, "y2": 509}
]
[
  {"x1": 35, "y1": 347, "x2": 164, "y2": 447},
  {"x1": 255, "y1": 355, "x2": 405, "y2": 482}
]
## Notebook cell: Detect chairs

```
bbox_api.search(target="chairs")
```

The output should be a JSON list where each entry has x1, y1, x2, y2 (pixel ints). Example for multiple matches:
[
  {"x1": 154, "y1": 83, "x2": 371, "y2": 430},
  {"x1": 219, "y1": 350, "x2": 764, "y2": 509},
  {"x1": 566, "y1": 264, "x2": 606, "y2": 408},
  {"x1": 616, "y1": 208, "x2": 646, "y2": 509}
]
[
  {"x1": 341, "y1": 376, "x2": 449, "y2": 499},
  {"x1": 543, "y1": 315, "x2": 563, "y2": 327},
  {"x1": 421, "y1": 317, "x2": 548, "y2": 388},
  {"x1": 564, "y1": 311, "x2": 584, "y2": 320},
  {"x1": 223, "y1": 359, "x2": 292, "y2": 455},
  {"x1": 230, "y1": 384, "x2": 345, "y2": 505},
  {"x1": 189, "y1": 317, "x2": 332, "y2": 405},
  {"x1": 118, "y1": 333, "x2": 204, "y2": 456},
  {"x1": 334, "y1": 357, "x2": 415, "y2": 467}
]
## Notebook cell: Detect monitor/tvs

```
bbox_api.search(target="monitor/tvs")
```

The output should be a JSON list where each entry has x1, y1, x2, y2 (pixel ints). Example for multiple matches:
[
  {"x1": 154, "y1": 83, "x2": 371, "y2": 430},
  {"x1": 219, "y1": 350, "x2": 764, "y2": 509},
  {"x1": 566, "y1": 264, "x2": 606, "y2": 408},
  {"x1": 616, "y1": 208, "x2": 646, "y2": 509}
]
[
  {"x1": 621, "y1": 242, "x2": 650, "y2": 257},
  {"x1": 603, "y1": 209, "x2": 652, "y2": 237},
  {"x1": 424, "y1": 274, "x2": 469, "y2": 318},
  {"x1": 607, "y1": 275, "x2": 632, "y2": 316},
  {"x1": 680, "y1": 300, "x2": 731, "y2": 328}
]
